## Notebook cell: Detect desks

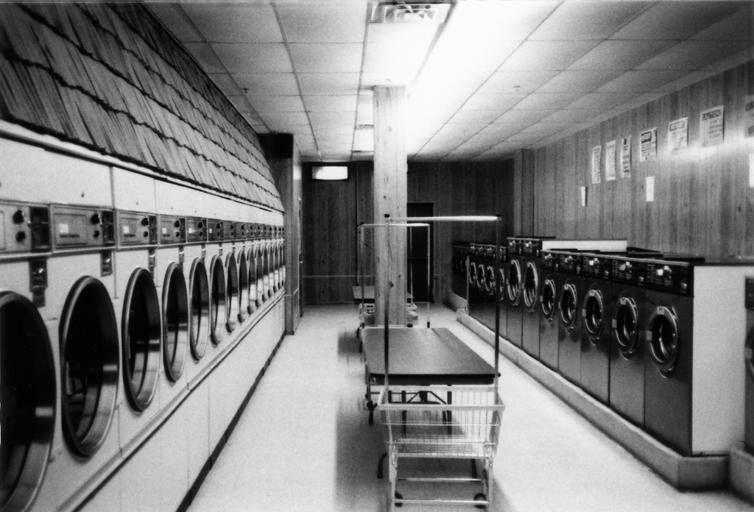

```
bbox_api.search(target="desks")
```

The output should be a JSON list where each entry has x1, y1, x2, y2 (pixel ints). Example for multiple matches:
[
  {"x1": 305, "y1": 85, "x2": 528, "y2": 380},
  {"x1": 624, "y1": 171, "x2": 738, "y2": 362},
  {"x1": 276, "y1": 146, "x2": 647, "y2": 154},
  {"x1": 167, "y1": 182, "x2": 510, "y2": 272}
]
[{"x1": 358, "y1": 327, "x2": 501, "y2": 439}]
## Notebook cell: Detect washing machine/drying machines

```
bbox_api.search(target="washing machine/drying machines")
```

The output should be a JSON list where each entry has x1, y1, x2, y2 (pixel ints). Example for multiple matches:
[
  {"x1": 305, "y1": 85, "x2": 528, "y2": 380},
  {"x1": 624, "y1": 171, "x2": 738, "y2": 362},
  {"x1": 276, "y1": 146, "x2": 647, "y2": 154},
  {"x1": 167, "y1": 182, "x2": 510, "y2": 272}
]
[
  {"x1": 451, "y1": 238, "x2": 754, "y2": 458},
  {"x1": 0, "y1": 119, "x2": 287, "y2": 511}
]
